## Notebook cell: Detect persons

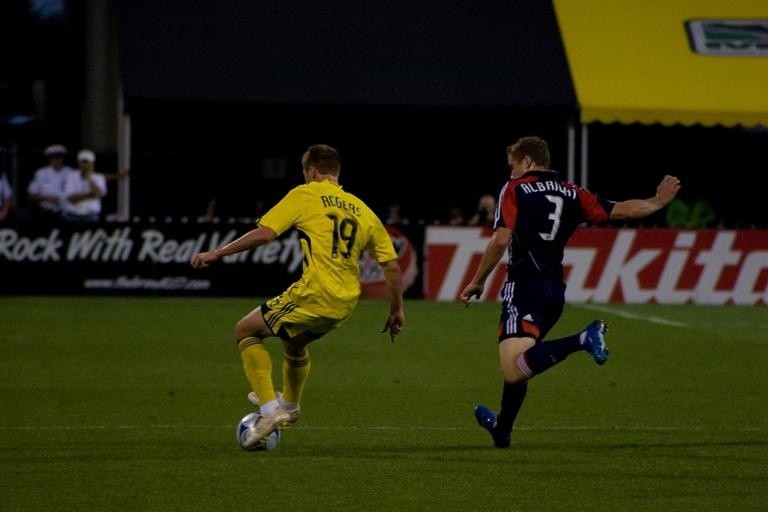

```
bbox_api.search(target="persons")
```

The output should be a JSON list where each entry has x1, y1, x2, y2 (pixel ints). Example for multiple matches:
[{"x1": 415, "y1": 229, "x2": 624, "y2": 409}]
[
  {"x1": 469, "y1": 195, "x2": 495, "y2": 225},
  {"x1": 385, "y1": 206, "x2": 408, "y2": 224},
  {"x1": 27, "y1": 145, "x2": 129, "y2": 220},
  {"x1": 460, "y1": 137, "x2": 682, "y2": 449},
  {"x1": 189, "y1": 143, "x2": 406, "y2": 449},
  {"x1": 0, "y1": 173, "x2": 15, "y2": 228},
  {"x1": 63, "y1": 149, "x2": 109, "y2": 220}
]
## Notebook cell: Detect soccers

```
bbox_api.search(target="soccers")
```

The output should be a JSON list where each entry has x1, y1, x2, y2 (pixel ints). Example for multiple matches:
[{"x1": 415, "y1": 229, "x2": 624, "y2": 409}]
[{"x1": 236, "y1": 411, "x2": 281, "y2": 452}]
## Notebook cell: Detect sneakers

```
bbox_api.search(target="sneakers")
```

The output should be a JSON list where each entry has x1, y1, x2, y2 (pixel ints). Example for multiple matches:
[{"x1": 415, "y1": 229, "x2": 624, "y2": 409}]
[
  {"x1": 247, "y1": 389, "x2": 301, "y2": 424},
  {"x1": 584, "y1": 319, "x2": 610, "y2": 366},
  {"x1": 241, "y1": 402, "x2": 291, "y2": 449},
  {"x1": 472, "y1": 404, "x2": 512, "y2": 449}
]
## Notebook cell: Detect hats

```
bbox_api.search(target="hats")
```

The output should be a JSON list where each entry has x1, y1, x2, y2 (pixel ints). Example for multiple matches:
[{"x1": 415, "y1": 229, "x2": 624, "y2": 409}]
[
  {"x1": 44, "y1": 145, "x2": 66, "y2": 155},
  {"x1": 77, "y1": 149, "x2": 96, "y2": 163}
]
